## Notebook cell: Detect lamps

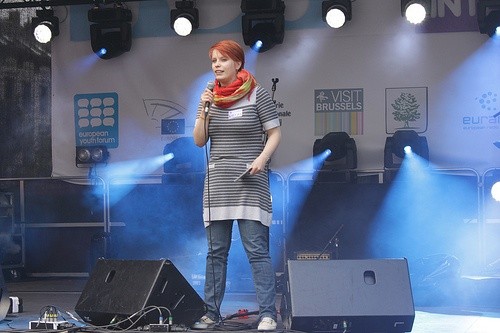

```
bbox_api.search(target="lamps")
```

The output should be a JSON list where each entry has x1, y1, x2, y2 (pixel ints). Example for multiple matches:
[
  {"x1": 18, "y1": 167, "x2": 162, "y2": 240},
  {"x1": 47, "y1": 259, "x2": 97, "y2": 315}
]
[
  {"x1": 75, "y1": 145, "x2": 109, "y2": 167},
  {"x1": 163, "y1": 135, "x2": 206, "y2": 174},
  {"x1": 475, "y1": 0, "x2": 500, "y2": 37},
  {"x1": 170, "y1": 0, "x2": 200, "y2": 37},
  {"x1": 384, "y1": 130, "x2": 430, "y2": 169},
  {"x1": 240, "y1": 0, "x2": 285, "y2": 52},
  {"x1": 32, "y1": 7, "x2": 59, "y2": 43},
  {"x1": 401, "y1": 0, "x2": 430, "y2": 23},
  {"x1": 88, "y1": 2, "x2": 133, "y2": 60},
  {"x1": 313, "y1": 131, "x2": 358, "y2": 170},
  {"x1": 322, "y1": 0, "x2": 352, "y2": 29}
]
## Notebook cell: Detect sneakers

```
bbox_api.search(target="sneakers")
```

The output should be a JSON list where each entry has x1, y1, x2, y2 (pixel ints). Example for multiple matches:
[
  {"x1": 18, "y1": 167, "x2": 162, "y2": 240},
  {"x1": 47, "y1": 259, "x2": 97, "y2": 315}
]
[
  {"x1": 192, "y1": 316, "x2": 221, "y2": 330},
  {"x1": 258, "y1": 317, "x2": 277, "y2": 331}
]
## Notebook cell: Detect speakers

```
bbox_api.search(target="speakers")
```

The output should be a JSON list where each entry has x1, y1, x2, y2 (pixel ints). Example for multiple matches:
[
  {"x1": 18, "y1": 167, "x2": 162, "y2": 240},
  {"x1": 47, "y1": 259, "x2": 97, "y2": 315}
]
[
  {"x1": 278, "y1": 258, "x2": 416, "y2": 333},
  {"x1": 0, "y1": 269, "x2": 11, "y2": 322},
  {"x1": 75, "y1": 258, "x2": 209, "y2": 330}
]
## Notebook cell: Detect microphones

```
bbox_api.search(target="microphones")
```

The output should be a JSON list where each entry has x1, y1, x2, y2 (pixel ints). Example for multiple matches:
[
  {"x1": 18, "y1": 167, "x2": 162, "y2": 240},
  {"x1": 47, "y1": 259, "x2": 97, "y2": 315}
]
[{"x1": 205, "y1": 81, "x2": 215, "y2": 116}]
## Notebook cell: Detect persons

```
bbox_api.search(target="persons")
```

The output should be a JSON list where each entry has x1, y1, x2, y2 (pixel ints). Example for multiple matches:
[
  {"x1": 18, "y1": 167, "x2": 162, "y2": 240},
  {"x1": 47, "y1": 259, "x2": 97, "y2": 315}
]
[{"x1": 191, "y1": 40, "x2": 282, "y2": 331}]
